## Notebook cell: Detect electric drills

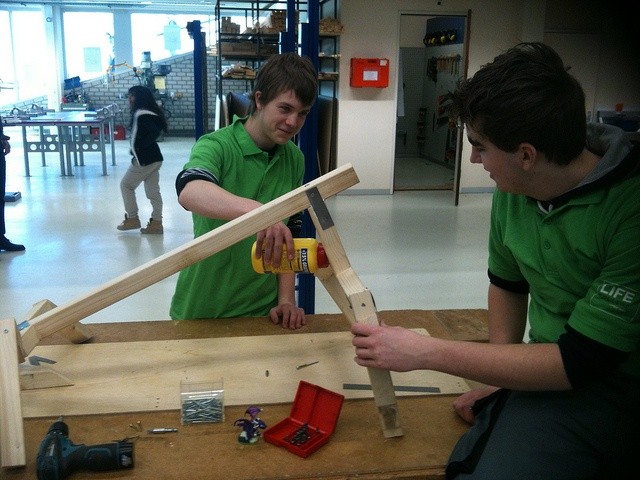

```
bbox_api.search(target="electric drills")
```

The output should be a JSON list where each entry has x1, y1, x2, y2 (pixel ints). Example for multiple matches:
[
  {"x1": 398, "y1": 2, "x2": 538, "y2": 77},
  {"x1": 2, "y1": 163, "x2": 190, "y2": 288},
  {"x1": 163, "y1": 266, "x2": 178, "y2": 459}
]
[{"x1": 36, "y1": 416, "x2": 136, "y2": 480}]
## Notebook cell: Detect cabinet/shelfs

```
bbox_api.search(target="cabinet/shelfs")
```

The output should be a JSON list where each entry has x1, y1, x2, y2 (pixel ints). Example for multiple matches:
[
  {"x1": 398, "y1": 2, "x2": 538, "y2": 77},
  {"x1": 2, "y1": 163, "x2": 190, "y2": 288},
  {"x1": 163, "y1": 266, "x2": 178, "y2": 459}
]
[{"x1": 214, "y1": 0, "x2": 339, "y2": 176}]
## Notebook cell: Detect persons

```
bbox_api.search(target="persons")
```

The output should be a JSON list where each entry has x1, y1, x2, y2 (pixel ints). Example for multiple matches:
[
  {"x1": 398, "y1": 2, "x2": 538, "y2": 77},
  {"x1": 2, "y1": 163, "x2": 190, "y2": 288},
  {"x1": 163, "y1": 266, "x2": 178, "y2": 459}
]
[
  {"x1": 166, "y1": 55, "x2": 309, "y2": 330},
  {"x1": 347, "y1": 38, "x2": 639, "y2": 480},
  {"x1": 117, "y1": 84, "x2": 165, "y2": 237},
  {"x1": 0, "y1": 114, "x2": 25, "y2": 252}
]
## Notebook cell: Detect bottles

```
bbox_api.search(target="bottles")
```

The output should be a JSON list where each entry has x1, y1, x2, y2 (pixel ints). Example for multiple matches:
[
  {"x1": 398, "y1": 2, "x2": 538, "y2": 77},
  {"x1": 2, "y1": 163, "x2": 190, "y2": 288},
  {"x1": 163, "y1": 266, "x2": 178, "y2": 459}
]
[{"x1": 251, "y1": 238, "x2": 329, "y2": 272}]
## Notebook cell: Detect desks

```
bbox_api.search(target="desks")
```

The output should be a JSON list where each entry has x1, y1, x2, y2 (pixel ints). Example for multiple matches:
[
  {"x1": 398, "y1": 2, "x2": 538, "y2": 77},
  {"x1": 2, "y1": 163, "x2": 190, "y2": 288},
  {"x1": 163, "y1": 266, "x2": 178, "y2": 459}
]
[
  {"x1": 1, "y1": 308, "x2": 525, "y2": 479},
  {"x1": 5, "y1": 103, "x2": 119, "y2": 177}
]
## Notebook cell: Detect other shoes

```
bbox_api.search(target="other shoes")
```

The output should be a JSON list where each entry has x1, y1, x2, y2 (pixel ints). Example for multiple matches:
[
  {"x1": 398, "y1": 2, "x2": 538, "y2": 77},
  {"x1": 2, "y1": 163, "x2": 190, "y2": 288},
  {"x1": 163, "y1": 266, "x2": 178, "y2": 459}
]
[{"x1": 0, "y1": 234, "x2": 25, "y2": 252}]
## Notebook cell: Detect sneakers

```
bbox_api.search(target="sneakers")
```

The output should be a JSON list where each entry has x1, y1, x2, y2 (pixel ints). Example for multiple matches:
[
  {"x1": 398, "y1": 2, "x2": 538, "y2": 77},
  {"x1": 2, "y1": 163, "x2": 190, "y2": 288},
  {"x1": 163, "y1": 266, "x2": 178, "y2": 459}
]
[
  {"x1": 140, "y1": 218, "x2": 163, "y2": 234},
  {"x1": 117, "y1": 213, "x2": 141, "y2": 230}
]
